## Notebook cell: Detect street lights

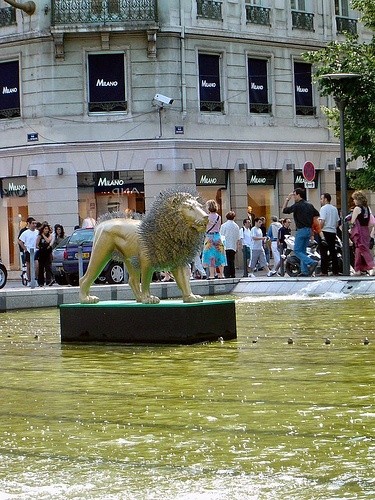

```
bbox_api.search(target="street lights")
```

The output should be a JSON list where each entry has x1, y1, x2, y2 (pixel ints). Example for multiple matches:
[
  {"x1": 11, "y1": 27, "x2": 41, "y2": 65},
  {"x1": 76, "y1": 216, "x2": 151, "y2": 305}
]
[{"x1": 320, "y1": 71, "x2": 363, "y2": 276}]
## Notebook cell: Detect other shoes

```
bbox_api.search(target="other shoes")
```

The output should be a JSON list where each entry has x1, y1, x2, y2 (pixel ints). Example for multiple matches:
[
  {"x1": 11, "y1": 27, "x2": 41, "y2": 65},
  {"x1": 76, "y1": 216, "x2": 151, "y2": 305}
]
[
  {"x1": 206, "y1": 276, "x2": 215, "y2": 280},
  {"x1": 218, "y1": 275, "x2": 225, "y2": 278},
  {"x1": 40, "y1": 285, "x2": 43, "y2": 287},
  {"x1": 49, "y1": 280, "x2": 55, "y2": 286},
  {"x1": 226, "y1": 275, "x2": 235, "y2": 278},
  {"x1": 274, "y1": 273, "x2": 280, "y2": 277},
  {"x1": 266, "y1": 271, "x2": 276, "y2": 277},
  {"x1": 309, "y1": 261, "x2": 317, "y2": 275},
  {"x1": 248, "y1": 272, "x2": 255, "y2": 277},
  {"x1": 350, "y1": 270, "x2": 361, "y2": 276},
  {"x1": 297, "y1": 273, "x2": 308, "y2": 276},
  {"x1": 370, "y1": 268, "x2": 375, "y2": 275},
  {"x1": 27, "y1": 280, "x2": 38, "y2": 287}
]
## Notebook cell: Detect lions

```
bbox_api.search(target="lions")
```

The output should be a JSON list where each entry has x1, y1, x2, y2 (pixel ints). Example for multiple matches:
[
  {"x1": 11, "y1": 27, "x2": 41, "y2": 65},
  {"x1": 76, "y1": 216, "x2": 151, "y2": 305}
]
[{"x1": 79, "y1": 185, "x2": 210, "y2": 305}]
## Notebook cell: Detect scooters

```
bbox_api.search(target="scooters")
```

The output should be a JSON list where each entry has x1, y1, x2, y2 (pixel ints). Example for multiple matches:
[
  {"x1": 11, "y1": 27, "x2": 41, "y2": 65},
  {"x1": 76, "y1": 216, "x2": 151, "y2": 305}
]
[{"x1": 281, "y1": 233, "x2": 351, "y2": 278}]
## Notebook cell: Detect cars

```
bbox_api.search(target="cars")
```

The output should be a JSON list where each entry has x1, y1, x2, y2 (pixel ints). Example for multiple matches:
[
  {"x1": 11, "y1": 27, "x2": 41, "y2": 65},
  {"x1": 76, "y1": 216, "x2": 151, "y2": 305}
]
[
  {"x1": 51, "y1": 235, "x2": 71, "y2": 286},
  {"x1": 63, "y1": 227, "x2": 125, "y2": 287}
]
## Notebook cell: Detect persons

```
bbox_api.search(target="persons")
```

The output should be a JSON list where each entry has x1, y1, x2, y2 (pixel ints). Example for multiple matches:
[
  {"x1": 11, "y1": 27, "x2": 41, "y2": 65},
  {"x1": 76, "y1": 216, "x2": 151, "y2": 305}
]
[
  {"x1": 18, "y1": 216, "x2": 81, "y2": 288},
  {"x1": 154, "y1": 188, "x2": 375, "y2": 282},
  {"x1": 125, "y1": 209, "x2": 144, "y2": 220}
]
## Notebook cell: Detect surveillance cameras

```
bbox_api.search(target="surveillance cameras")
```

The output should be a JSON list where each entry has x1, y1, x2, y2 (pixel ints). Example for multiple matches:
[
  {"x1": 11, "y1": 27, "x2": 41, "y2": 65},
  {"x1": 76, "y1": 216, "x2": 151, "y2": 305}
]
[{"x1": 153, "y1": 93, "x2": 174, "y2": 105}]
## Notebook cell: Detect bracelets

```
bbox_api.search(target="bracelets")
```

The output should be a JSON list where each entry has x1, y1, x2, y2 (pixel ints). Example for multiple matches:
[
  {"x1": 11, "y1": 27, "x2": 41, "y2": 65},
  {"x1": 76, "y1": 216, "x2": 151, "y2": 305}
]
[{"x1": 285, "y1": 197, "x2": 289, "y2": 201}]
